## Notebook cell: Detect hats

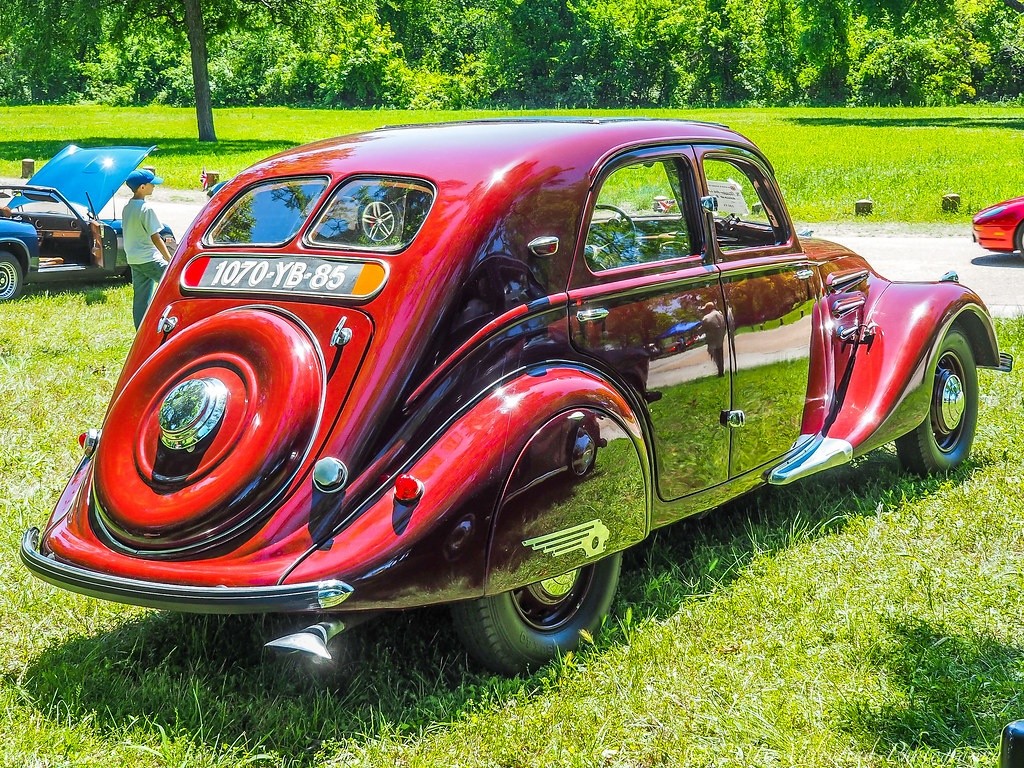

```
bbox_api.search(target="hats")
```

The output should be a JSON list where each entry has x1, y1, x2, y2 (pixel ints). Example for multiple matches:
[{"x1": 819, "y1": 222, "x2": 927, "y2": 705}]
[
  {"x1": 701, "y1": 302, "x2": 714, "y2": 309},
  {"x1": 126, "y1": 169, "x2": 164, "y2": 192}
]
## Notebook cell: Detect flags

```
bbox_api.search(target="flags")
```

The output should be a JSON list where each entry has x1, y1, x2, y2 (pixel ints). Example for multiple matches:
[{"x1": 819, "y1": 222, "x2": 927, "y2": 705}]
[
  {"x1": 200, "y1": 170, "x2": 208, "y2": 190},
  {"x1": 660, "y1": 200, "x2": 675, "y2": 210}
]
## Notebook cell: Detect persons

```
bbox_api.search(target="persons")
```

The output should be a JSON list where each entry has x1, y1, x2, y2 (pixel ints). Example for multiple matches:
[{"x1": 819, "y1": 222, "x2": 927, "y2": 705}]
[
  {"x1": 123, "y1": 170, "x2": 172, "y2": 331},
  {"x1": 698, "y1": 303, "x2": 726, "y2": 377}
]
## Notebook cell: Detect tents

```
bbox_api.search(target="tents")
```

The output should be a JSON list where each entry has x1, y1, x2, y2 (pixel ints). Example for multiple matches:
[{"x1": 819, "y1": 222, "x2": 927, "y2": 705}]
[{"x1": 658, "y1": 321, "x2": 699, "y2": 342}]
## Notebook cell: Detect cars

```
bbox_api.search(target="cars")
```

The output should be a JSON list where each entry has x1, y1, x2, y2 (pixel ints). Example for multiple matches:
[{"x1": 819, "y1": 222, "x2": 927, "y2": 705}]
[
  {"x1": 971, "y1": 196, "x2": 1024, "y2": 258},
  {"x1": 0, "y1": 144, "x2": 178, "y2": 302},
  {"x1": 21, "y1": 118, "x2": 1016, "y2": 681}
]
